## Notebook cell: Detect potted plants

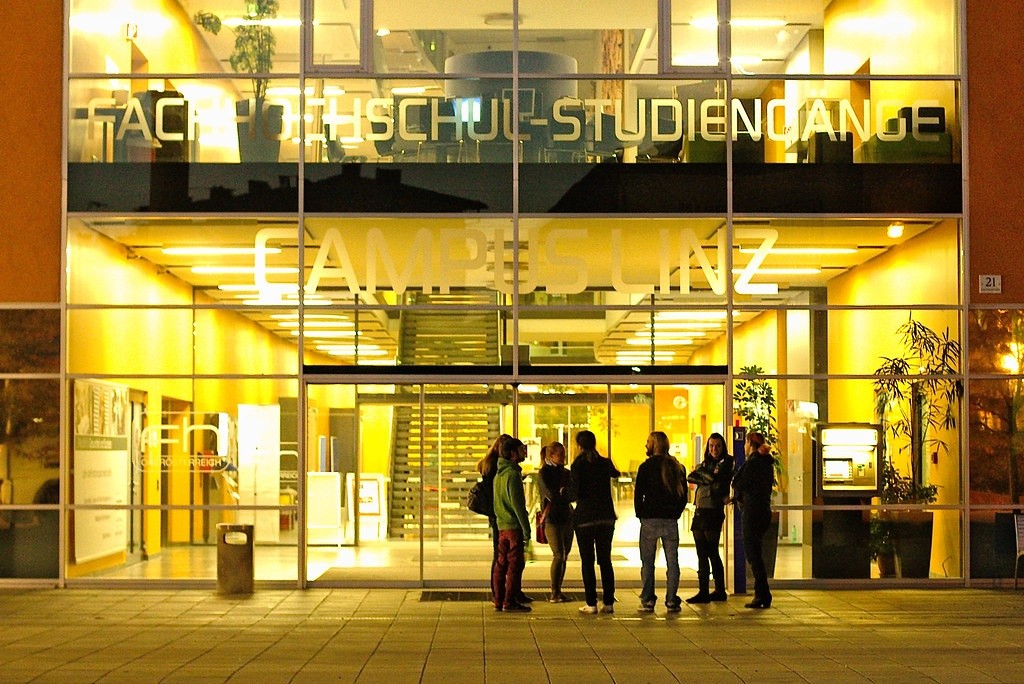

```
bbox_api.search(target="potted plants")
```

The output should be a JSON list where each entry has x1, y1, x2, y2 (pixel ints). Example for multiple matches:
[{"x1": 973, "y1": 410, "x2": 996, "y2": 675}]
[
  {"x1": 868, "y1": 309, "x2": 964, "y2": 579},
  {"x1": 195, "y1": 0, "x2": 283, "y2": 163},
  {"x1": 732, "y1": 366, "x2": 783, "y2": 577}
]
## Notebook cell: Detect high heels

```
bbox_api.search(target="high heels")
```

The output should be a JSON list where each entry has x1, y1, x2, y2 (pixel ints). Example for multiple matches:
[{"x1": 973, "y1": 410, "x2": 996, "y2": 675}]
[{"x1": 745, "y1": 596, "x2": 772, "y2": 608}]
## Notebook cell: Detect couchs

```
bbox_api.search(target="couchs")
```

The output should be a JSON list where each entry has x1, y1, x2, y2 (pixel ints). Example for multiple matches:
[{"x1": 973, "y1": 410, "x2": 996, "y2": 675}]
[
  {"x1": 685, "y1": 99, "x2": 765, "y2": 162},
  {"x1": 785, "y1": 107, "x2": 954, "y2": 164}
]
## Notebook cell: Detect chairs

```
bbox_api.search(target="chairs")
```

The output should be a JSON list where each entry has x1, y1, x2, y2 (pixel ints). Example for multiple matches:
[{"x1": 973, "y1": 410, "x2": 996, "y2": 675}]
[
  {"x1": 372, "y1": 101, "x2": 683, "y2": 163},
  {"x1": 324, "y1": 124, "x2": 367, "y2": 163},
  {"x1": 112, "y1": 91, "x2": 189, "y2": 163},
  {"x1": 1014, "y1": 515, "x2": 1024, "y2": 590}
]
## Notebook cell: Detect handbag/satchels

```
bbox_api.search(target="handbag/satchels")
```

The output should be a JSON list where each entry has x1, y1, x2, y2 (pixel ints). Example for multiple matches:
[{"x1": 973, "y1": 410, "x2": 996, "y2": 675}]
[
  {"x1": 466, "y1": 481, "x2": 493, "y2": 517},
  {"x1": 536, "y1": 511, "x2": 550, "y2": 543}
]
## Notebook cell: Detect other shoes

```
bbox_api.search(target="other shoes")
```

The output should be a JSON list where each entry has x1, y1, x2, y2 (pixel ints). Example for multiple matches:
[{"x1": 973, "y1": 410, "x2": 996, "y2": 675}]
[
  {"x1": 491, "y1": 597, "x2": 498, "y2": 605},
  {"x1": 519, "y1": 593, "x2": 534, "y2": 603},
  {"x1": 685, "y1": 592, "x2": 711, "y2": 603},
  {"x1": 710, "y1": 591, "x2": 727, "y2": 601},
  {"x1": 549, "y1": 596, "x2": 573, "y2": 602},
  {"x1": 494, "y1": 603, "x2": 502, "y2": 610},
  {"x1": 502, "y1": 602, "x2": 531, "y2": 612}
]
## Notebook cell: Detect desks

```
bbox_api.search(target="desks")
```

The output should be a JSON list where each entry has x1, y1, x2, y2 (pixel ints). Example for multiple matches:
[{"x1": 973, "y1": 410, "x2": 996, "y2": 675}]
[{"x1": 70, "y1": 108, "x2": 140, "y2": 164}]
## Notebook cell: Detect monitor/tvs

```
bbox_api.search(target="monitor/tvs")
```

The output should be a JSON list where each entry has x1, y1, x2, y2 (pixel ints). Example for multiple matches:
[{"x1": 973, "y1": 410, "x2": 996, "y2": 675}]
[{"x1": 823, "y1": 458, "x2": 853, "y2": 479}]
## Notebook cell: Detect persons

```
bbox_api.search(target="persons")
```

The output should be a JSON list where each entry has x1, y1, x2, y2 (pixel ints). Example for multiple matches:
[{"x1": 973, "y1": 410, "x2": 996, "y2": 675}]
[
  {"x1": 633, "y1": 431, "x2": 688, "y2": 613},
  {"x1": 687, "y1": 431, "x2": 732, "y2": 604},
  {"x1": 555, "y1": 429, "x2": 621, "y2": 615},
  {"x1": 476, "y1": 433, "x2": 535, "y2": 604},
  {"x1": 492, "y1": 437, "x2": 532, "y2": 612},
  {"x1": 729, "y1": 431, "x2": 776, "y2": 610},
  {"x1": 534, "y1": 441, "x2": 574, "y2": 604}
]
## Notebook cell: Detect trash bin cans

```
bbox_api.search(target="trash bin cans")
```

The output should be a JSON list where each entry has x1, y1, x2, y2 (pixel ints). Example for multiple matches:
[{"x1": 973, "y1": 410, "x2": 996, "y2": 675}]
[{"x1": 215, "y1": 523, "x2": 254, "y2": 600}]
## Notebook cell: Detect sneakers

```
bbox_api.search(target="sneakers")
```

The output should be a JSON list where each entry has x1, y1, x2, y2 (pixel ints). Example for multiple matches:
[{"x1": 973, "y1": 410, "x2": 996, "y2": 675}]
[
  {"x1": 667, "y1": 605, "x2": 681, "y2": 612},
  {"x1": 600, "y1": 604, "x2": 613, "y2": 613},
  {"x1": 578, "y1": 604, "x2": 597, "y2": 614},
  {"x1": 637, "y1": 603, "x2": 654, "y2": 612}
]
